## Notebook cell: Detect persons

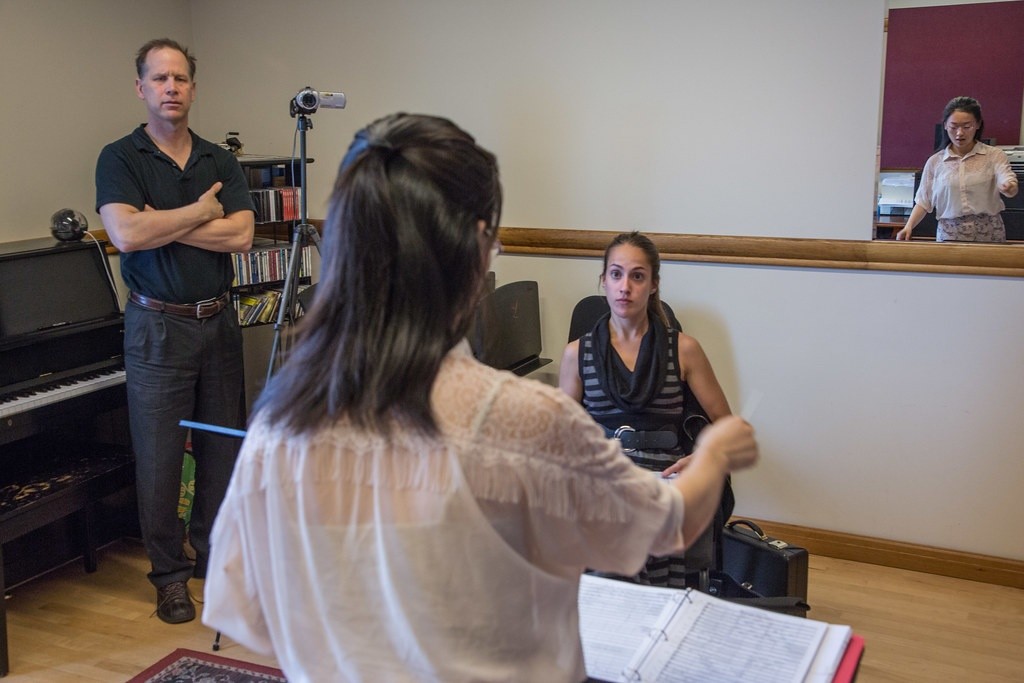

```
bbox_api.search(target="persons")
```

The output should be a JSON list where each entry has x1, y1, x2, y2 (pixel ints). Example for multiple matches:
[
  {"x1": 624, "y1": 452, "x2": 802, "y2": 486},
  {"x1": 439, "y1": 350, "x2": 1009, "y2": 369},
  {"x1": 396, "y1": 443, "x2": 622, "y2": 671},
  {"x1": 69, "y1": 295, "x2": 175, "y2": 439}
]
[
  {"x1": 95, "y1": 39, "x2": 259, "y2": 623},
  {"x1": 896, "y1": 96, "x2": 1019, "y2": 242},
  {"x1": 559, "y1": 234, "x2": 732, "y2": 476},
  {"x1": 202, "y1": 112, "x2": 758, "y2": 683}
]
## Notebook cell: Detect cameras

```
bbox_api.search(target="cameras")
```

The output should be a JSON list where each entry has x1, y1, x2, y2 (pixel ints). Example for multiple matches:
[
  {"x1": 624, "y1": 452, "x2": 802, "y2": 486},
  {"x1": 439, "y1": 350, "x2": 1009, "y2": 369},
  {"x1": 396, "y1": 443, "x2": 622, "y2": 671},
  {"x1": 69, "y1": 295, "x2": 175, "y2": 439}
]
[{"x1": 290, "y1": 86, "x2": 346, "y2": 118}]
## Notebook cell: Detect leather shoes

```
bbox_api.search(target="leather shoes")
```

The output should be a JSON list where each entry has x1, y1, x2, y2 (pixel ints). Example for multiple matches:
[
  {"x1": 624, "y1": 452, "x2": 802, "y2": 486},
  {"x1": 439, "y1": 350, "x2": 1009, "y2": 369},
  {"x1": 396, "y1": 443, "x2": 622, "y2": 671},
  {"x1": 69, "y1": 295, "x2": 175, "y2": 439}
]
[
  {"x1": 195, "y1": 551, "x2": 207, "y2": 579},
  {"x1": 156, "y1": 581, "x2": 196, "y2": 624}
]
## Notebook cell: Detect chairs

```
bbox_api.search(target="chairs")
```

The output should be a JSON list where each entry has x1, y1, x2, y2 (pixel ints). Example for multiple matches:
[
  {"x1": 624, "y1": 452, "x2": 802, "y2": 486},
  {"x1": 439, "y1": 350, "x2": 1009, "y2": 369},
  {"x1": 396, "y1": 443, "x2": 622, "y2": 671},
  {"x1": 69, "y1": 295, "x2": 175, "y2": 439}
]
[{"x1": 568, "y1": 296, "x2": 683, "y2": 343}]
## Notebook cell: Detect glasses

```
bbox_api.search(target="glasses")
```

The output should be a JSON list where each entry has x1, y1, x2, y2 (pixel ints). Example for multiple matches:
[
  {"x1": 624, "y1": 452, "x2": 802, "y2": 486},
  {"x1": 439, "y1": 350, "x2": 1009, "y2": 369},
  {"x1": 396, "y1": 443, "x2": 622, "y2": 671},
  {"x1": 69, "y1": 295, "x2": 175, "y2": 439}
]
[
  {"x1": 945, "y1": 122, "x2": 977, "y2": 132},
  {"x1": 486, "y1": 228, "x2": 502, "y2": 263}
]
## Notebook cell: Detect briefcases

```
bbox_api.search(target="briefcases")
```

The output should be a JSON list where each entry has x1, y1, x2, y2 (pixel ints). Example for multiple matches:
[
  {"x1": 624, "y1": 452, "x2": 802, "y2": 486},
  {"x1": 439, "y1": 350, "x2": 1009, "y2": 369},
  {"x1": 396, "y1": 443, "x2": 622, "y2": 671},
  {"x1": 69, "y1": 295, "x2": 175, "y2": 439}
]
[{"x1": 719, "y1": 518, "x2": 808, "y2": 618}]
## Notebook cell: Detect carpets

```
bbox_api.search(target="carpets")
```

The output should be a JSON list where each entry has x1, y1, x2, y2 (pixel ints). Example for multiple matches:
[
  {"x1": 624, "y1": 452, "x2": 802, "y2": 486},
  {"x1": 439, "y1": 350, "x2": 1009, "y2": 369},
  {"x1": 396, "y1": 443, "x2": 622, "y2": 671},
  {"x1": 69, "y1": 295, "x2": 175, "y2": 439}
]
[{"x1": 126, "y1": 648, "x2": 286, "y2": 683}]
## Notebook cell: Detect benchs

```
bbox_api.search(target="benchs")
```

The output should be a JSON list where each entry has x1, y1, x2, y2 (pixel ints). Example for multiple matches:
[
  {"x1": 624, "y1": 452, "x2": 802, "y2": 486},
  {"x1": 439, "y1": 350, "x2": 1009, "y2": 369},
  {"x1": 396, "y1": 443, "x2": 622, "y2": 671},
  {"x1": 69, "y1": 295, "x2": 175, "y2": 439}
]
[{"x1": 478, "y1": 281, "x2": 553, "y2": 377}]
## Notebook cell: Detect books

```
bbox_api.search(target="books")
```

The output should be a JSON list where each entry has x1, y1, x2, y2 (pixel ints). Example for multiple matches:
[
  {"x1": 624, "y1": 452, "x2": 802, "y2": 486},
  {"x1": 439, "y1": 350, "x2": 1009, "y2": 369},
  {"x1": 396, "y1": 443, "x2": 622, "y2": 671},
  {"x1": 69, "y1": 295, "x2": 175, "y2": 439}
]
[
  {"x1": 230, "y1": 243, "x2": 311, "y2": 327},
  {"x1": 249, "y1": 186, "x2": 301, "y2": 223}
]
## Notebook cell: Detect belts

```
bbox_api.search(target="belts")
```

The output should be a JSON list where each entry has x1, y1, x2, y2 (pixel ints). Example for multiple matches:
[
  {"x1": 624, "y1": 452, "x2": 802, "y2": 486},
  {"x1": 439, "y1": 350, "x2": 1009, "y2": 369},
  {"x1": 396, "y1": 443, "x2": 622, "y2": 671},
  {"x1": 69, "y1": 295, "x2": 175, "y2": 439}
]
[
  {"x1": 597, "y1": 423, "x2": 682, "y2": 452},
  {"x1": 128, "y1": 292, "x2": 231, "y2": 319}
]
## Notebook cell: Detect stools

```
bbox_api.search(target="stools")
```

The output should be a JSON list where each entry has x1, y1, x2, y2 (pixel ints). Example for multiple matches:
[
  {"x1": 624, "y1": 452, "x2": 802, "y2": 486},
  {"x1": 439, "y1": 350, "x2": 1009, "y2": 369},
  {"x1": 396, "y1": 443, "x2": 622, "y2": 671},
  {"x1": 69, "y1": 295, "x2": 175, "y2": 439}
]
[{"x1": 0, "y1": 440, "x2": 136, "y2": 677}]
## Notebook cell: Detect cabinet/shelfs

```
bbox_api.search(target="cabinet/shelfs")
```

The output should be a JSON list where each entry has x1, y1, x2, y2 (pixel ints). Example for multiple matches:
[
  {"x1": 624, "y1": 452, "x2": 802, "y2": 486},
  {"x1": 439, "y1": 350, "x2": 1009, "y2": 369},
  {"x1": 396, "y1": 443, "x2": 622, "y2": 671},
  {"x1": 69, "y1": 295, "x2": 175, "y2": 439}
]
[{"x1": 230, "y1": 154, "x2": 313, "y2": 329}]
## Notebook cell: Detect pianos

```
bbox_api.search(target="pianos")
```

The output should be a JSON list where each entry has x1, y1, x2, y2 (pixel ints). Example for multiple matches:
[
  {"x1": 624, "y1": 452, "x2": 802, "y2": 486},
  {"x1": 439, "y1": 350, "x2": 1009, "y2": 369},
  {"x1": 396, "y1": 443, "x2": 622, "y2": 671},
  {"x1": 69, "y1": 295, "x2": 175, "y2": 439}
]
[{"x1": 0, "y1": 236, "x2": 137, "y2": 595}]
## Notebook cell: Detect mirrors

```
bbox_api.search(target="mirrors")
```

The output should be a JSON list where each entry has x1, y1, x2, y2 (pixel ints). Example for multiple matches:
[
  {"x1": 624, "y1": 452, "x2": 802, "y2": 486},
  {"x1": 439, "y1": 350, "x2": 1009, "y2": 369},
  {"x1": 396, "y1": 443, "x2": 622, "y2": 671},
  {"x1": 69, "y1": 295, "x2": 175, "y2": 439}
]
[{"x1": 871, "y1": 0, "x2": 1023, "y2": 246}]
